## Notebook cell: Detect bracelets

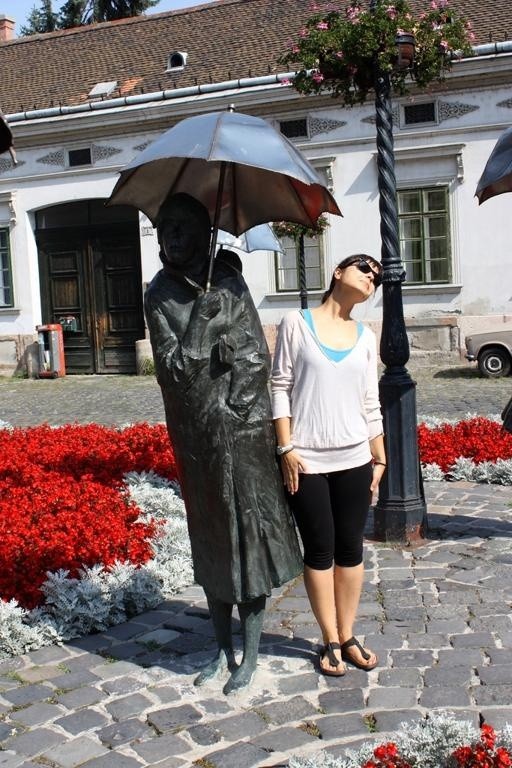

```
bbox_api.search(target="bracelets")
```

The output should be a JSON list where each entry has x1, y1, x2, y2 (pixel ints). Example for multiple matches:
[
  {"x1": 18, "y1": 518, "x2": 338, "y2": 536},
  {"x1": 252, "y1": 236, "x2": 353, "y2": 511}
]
[{"x1": 374, "y1": 462, "x2": 387, "y2": 467}]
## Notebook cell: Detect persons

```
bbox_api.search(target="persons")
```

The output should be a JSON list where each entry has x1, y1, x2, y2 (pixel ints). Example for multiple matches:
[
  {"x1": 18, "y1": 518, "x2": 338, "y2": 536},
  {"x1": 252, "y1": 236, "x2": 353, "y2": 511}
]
[
  {"x1": 271, "y1": 254, "x2": 386, "y2": 676},
  {"x1": 145, "y1": 191, "x2": 308, "y2": 696}
]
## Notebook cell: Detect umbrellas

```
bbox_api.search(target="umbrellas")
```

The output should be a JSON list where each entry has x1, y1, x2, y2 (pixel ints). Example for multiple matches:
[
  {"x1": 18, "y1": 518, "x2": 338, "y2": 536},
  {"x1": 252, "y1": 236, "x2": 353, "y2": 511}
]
[
  {"x1": 472, "y1": 128, "x2": 512, "y2": 205},
  {"x1": 106, "y1": 103, "x2": 345, "y2": 298}
]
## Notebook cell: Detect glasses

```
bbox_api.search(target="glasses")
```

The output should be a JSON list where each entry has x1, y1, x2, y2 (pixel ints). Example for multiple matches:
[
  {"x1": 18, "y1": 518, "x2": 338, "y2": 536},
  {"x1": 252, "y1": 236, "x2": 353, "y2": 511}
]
[{"x1": 348, "y1": 260, "x2": 382, "y2": 288}]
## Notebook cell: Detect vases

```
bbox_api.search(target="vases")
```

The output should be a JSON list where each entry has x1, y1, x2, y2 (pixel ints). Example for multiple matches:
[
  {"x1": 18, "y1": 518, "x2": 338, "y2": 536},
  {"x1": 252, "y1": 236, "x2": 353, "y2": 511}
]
[{"x1": 380, "y1": 34, "x2": 416, "y2": 68}]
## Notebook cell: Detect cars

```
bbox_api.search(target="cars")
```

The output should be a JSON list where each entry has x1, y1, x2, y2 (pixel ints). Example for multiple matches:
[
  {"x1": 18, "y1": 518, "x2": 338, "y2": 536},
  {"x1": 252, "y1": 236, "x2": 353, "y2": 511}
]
[{"x1": 464, "y1": 324, "x2": 512, "y2": 379}]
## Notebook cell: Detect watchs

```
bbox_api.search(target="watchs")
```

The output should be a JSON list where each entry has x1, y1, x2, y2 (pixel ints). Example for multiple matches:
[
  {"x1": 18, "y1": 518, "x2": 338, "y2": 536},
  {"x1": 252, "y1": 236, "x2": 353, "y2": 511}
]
[{"x1": 275, "y1": 446, "x2": 294, "y2": 455}]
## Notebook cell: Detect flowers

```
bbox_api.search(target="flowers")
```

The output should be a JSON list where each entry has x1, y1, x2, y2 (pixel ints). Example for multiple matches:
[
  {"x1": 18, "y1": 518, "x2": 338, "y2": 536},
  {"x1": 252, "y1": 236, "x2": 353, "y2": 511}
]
[{"x1": 269, "y1": 1, "x2": 478, "y2": 110}]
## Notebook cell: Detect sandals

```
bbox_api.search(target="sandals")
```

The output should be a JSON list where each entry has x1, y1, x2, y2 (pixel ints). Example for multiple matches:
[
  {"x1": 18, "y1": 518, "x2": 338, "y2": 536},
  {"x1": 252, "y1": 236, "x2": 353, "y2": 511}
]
[{"x1": 318, "y1": 635, "x2": 379, "y2": 676}]
